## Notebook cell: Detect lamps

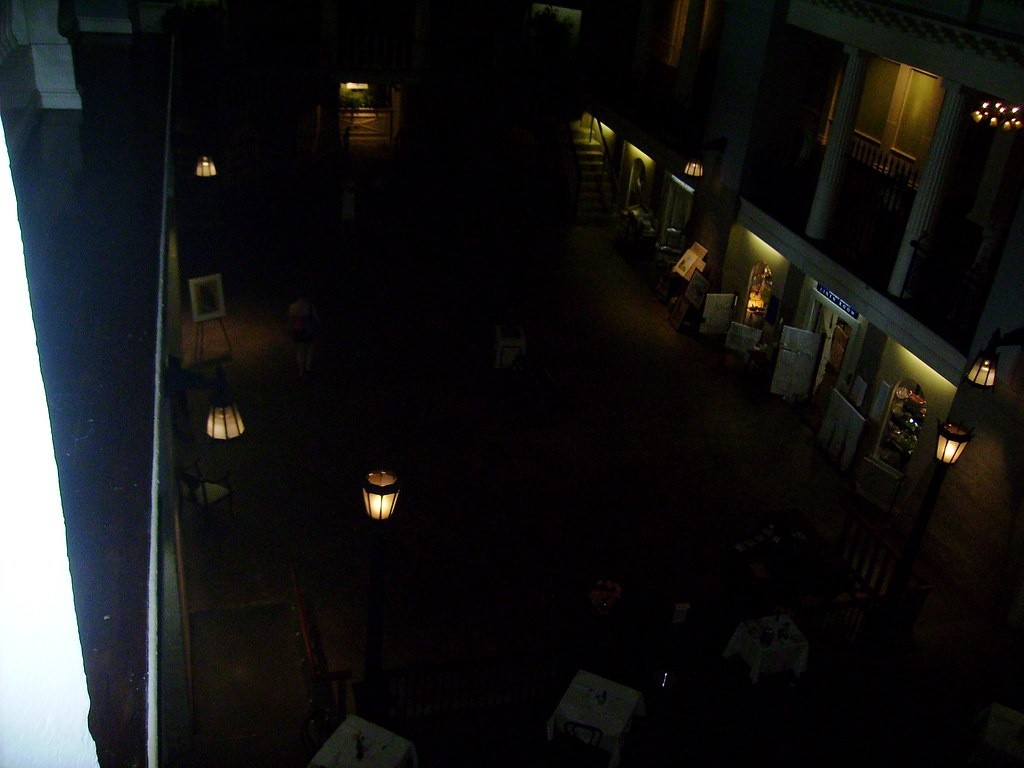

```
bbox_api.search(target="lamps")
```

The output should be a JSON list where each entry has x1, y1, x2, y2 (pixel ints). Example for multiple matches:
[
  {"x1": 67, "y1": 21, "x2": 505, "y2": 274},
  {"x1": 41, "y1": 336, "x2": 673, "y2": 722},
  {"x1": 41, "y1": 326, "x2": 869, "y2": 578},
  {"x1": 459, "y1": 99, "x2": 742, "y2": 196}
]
[
  {"x1": 357, "y1": 409, "x2": 400, "y2": 520},
  {"x1": 684, "y1": 142, "x2": 704, "y2": 177},
  {"x1": 195, "y1": 150, "x2": 216, "y2": 177},
  {"x1": 205, "y1": 364, "x2": 245, "y2": 441},
  {"x1": 936, "y1": 418, "x2": 977, "y2": 465},
  {"x1": 967, "y1": 328, "x2": 1001, "y2": 387}
]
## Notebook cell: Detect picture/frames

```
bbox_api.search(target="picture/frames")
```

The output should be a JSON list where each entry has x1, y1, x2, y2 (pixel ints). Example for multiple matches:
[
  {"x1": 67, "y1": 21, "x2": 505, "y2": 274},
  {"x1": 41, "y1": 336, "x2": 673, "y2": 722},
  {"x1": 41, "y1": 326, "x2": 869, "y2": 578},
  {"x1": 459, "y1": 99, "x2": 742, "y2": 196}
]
[{"x1": 188, "y1": 274, "x2": 225, "y2": 322}]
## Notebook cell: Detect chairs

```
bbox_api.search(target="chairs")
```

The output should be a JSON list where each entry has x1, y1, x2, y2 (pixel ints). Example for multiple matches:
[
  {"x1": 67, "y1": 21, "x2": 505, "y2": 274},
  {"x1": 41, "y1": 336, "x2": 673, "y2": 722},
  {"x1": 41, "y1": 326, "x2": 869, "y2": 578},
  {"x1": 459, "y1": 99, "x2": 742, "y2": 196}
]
[
  {"x1": 172, "y1": 458, "x2": 235, "y2": 527},
  {"x1": 563, "y1": 721, "x2": 603, "y2": 768},
  {"x1": 303, "y1": 709, "x2": 332, "y2": 753}
]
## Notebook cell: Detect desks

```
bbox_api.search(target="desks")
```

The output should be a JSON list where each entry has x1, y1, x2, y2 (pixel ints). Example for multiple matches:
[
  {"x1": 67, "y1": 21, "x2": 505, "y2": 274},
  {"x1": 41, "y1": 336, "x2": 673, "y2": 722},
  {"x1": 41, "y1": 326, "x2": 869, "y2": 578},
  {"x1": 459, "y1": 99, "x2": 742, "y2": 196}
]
[
  {"x1": 960, "y1": 703, "x2": 1024, "y2": 768},
  {"x1": 547, "y1": 668, "x2": 640, "y2": 768},
  {"x1": 722, "y1": 614, "x2": 809, "y2": 686},
  {"x1": 307, "y1": 713, "x2": 418, "y2": 768},
  {"x1": 490, "y1": 325, "x2": 526, "y2": 370}
]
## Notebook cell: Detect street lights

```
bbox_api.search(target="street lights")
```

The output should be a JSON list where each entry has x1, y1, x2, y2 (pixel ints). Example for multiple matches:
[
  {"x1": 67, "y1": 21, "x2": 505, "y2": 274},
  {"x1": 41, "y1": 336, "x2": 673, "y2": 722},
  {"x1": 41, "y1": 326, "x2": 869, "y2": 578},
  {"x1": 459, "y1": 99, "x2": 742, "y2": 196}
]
[
  {"x1": 880, "y1": 420, "x2": 974, "y2": 635},
  {"x1": 356, "y1": 469, "x2": 403, "y2": 725}
]
